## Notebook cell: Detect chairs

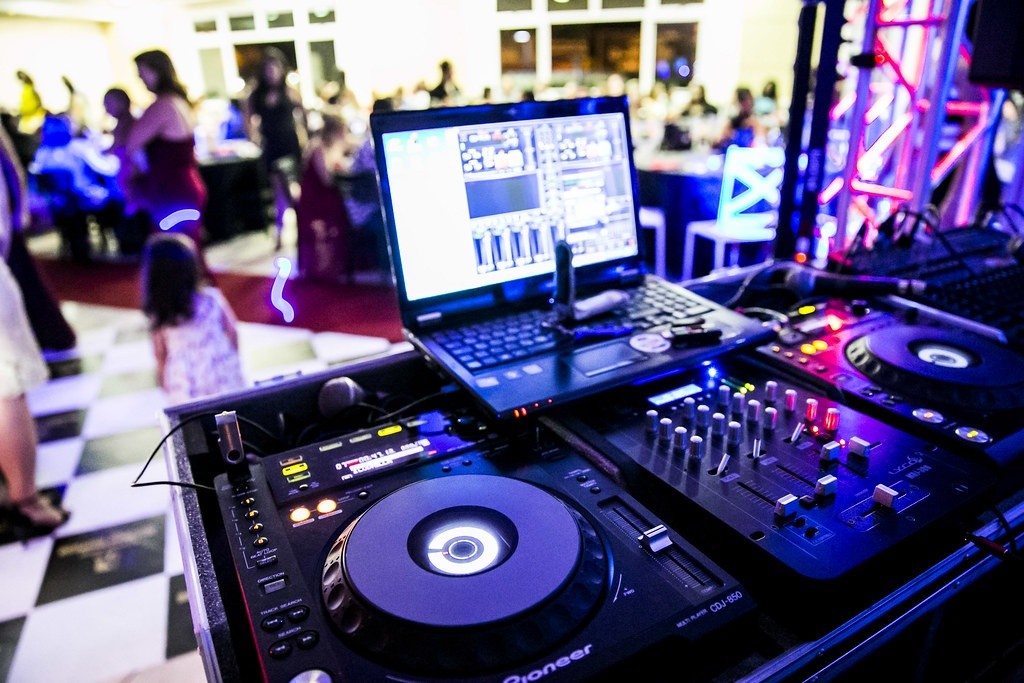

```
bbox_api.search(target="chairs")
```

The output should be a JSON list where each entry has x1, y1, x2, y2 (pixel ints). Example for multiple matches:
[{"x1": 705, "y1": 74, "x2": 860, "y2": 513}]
[{"x1": 682, "y1": 144, "x2": 787, "y2": 279}]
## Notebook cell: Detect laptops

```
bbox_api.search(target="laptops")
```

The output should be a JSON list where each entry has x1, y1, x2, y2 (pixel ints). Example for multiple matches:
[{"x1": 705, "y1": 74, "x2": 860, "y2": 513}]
[{"x1": 369, "y1": 96, "x2": 776, "y2": 417}]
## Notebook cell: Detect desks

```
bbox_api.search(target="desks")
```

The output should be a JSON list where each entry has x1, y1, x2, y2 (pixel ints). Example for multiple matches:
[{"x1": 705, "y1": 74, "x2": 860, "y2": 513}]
[{"x1": 633, "y1": 147, "x2": 785, "y2": 282}]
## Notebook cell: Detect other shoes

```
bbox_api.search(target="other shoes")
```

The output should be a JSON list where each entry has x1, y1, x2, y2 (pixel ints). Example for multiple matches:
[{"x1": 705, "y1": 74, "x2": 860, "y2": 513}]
[{"x1": 273, "y1": 243, "x2": 282, "y2": 252}]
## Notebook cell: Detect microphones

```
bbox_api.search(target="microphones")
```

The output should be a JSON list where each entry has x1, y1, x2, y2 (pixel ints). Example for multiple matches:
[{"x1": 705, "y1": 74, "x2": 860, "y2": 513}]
[
  {"x1": 785, "y1": 267, "x2": 927, "y2": 299},
  {"x1": 320, "y1": 377, "x2": 430, "y2": 421}
]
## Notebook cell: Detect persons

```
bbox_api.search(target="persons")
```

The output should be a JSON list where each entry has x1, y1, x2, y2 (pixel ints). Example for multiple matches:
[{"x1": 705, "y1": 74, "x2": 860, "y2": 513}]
[
  {"x1": 138, "y1": 234, "x2": 246, "y2": 404},
  {"x1": 103, "y1": 47, "x2": 218, "y2": 288},
  {"x1": 0, "y1": 117, "x2": 76, "y2": 543},
  {"x1": 244, "y1": 46, "x2": 778, "y2": 217}
]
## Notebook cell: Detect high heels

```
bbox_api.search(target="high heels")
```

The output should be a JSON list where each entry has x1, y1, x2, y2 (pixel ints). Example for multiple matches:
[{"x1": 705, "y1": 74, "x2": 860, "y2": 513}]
[{"x1": 1, "y1": 493, "x2": 72, "y2": 548}]
